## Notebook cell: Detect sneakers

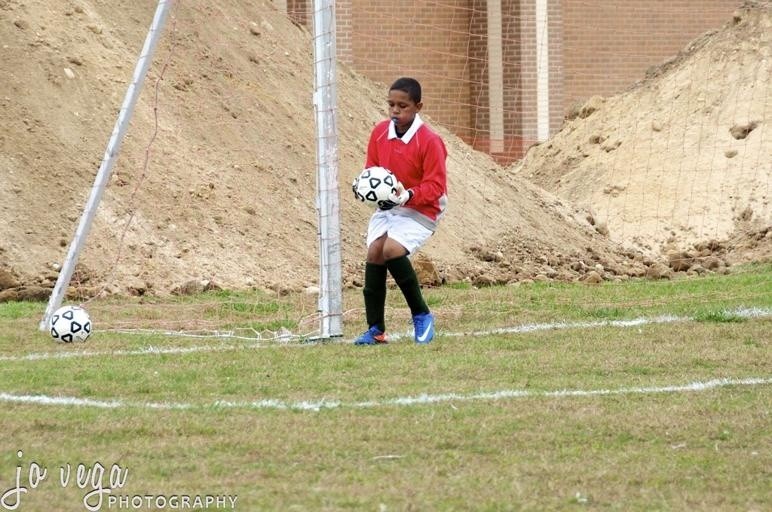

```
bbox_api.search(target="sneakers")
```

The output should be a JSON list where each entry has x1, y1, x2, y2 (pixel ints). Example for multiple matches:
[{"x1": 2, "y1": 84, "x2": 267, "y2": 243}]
[
  {"x1": 408, "y1": 312, "x2": 435, "y2": 345},
  {"x1": 353, "y1": 324, "x2": 386, "y2": 345}
]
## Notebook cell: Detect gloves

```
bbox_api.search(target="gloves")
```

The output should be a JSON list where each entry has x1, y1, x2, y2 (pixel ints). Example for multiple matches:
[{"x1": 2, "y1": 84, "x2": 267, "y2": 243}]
[
  {"x1": 377, "y1": 179, "x2": 413, "y2": 212},
  {"x1": 352, "y1": 177, "x2": 358, "y2": 199}
]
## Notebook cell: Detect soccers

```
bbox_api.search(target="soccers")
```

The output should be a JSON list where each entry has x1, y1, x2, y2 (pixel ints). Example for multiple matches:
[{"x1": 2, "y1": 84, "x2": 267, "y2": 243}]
[
  {"x1": 356, "y1": 167, "x2": 397, "y2": 208},
  {"x1": 50, "y1": 306, "x2": 91, "y2": 343}
]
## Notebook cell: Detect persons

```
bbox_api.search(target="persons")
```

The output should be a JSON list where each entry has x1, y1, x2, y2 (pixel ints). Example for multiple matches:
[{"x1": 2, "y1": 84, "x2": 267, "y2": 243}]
[{"x1": 353, "y1": 77, "x2": 447, "y2": 345}]
